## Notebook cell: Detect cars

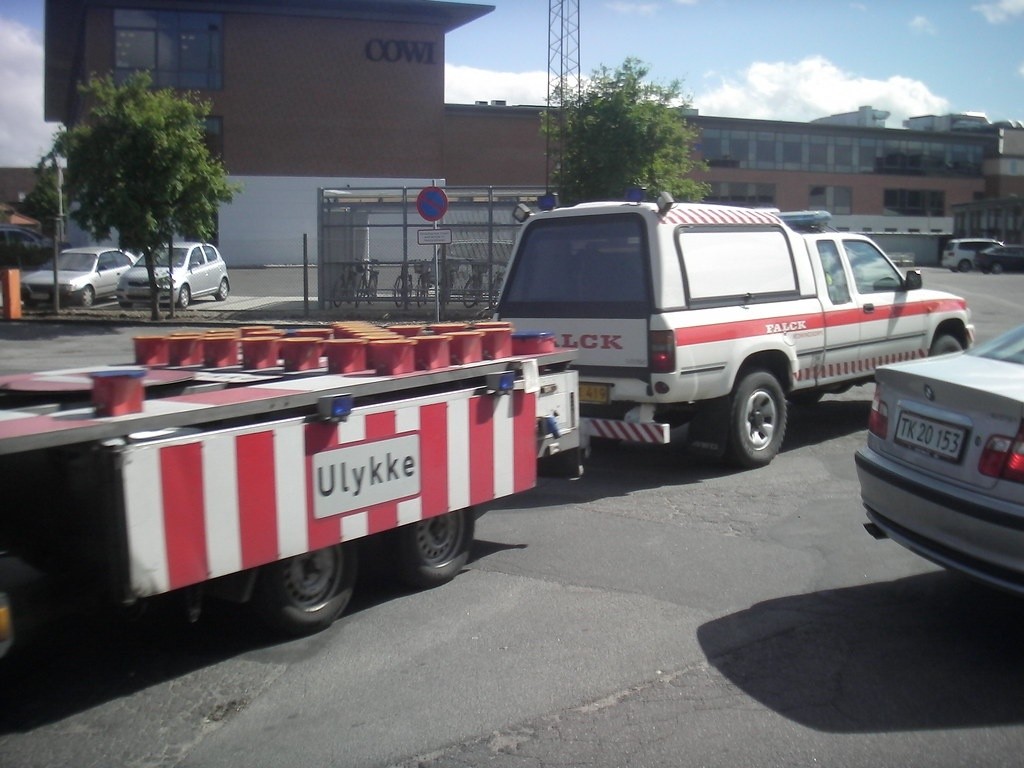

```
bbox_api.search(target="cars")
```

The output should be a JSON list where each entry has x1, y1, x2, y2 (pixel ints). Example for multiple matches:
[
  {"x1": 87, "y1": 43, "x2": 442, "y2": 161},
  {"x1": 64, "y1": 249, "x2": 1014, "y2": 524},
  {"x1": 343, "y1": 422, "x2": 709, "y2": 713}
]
[
  {"x1": 117, "y1": 242, "x2": 230, "y2": 308},
  {"x1": 0, "y1": 225, "x2": 72, "y2": 295},
  {"x1": 974, "y1": 245, "x2": 1023, "y2": 274},
  {"x1": 854, "y1": 318, "x2": 1024, "y2": 597},
  {"x1": 21, "y1": 247, "x2": 138, "y2": 308}
]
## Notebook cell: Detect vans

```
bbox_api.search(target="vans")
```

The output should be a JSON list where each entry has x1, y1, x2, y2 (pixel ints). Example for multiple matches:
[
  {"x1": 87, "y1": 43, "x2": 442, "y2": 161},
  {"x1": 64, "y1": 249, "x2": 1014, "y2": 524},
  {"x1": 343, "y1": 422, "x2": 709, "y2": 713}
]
[{"x1": 942, "y1": 238, "x2": 1004, "y2": 273}]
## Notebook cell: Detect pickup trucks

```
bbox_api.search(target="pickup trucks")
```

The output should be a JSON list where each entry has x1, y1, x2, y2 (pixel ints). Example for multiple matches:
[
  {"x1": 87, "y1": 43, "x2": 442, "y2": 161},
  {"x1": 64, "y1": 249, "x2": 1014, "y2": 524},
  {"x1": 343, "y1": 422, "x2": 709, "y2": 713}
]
[{"x1": 492, "y1": 187, "x2": 976, "y2": 470}]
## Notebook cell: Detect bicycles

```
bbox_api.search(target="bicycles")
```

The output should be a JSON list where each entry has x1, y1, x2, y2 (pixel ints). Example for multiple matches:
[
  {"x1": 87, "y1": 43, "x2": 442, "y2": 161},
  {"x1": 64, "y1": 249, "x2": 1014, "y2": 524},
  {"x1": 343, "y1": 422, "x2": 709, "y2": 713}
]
[
  {"x1": 331, "y1": 259, "x2": 380, "y2": 308},
  {"x1": 392, "y1": 259, "x2": 416, "y2": 305},
  {"x1": 413, "y1": 260, "x2": 436, "y2": 306},
  {"x1": 439, "y1": 259, "x2": 508, "y2": 308}
]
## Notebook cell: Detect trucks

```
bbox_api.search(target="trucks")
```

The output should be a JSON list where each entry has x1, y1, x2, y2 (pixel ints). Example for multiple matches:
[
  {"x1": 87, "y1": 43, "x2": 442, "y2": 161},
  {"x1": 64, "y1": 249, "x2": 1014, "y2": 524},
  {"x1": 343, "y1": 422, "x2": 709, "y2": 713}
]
[{"x1": 2, "y1": 345, "x2": 584, "y2": 661}]
